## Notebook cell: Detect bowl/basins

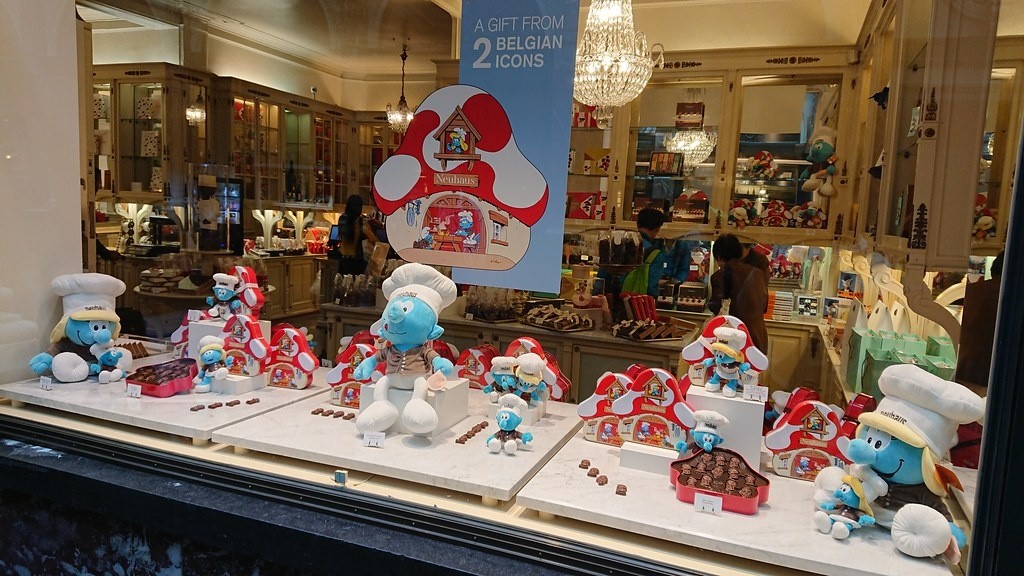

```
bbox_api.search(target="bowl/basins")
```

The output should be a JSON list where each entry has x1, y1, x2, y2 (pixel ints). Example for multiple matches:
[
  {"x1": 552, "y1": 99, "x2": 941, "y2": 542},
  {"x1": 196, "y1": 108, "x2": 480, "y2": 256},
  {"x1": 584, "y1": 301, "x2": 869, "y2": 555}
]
[{"x1": 130, "y1": 244, "x2": 180, "y2": 257}]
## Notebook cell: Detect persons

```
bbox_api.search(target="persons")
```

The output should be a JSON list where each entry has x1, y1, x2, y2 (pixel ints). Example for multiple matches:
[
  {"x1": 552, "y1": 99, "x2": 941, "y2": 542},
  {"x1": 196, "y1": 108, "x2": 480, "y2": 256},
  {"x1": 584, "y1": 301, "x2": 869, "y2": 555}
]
[
  {"x1": 709, "y1": 232, "x2": 770, "y2": 356},
  {"x1": 654, "y1": 235, "x2": 689, "y2": 285},
  {"x1": 594, "y1": 209, "x2": 664, "y2": 307},
  {"x1": 196, "y1": 187, "x2": 220, "y2": 251},
  {"x1": 337, "y1": 195, "x2": 395, "y2": 276},
  {"x1": 738, "y1": 240, "x2": 771, "y2": 286}
]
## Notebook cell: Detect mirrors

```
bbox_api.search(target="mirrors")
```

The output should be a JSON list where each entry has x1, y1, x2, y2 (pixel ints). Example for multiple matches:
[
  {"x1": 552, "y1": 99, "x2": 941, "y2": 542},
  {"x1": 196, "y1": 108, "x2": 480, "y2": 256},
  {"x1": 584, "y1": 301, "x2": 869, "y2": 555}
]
[
  {"x1": 615, "y1": 76, "x2": 727, "y2": 229},
  {"x1": 724, "y1": 67, "x2": 850, "y2": 236}
]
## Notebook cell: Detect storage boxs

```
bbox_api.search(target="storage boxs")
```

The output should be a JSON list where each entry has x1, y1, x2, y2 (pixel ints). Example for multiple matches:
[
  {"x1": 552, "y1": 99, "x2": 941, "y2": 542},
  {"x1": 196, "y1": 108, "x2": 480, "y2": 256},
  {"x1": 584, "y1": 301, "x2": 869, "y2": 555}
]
[
  {"x1": 568, "y1": 206, "x2": 591, "y2": 219},
  {"x1": 685, "y1": 384, "x2": 765, "y2": 474},
  {"x1": 657, "y1": 314, "x2": 697, "y2": 331},
  {"x1": 657, "y1": 281, "x2": 683, "y2": 310},
  {"x1": 658, "y1": 324, "x2": 676, "y2": 338},
  {"x1": 188, "y1": 317, "x2": 271, "y2": 384},
  {"x1": 595, "y1": 189, "x2": 607, "y2": 206},
  {"x1": 670, "y1": 329, "x2": 687, "y2": 338},
  {"x1": 649, "y1": 321, "x2": 667, "y2": 339},
  {"x1": 204, "y1": 372, "x2": 268, "y2": 396},
  {"x1": 570, "y1": 191, "x2": 596, "y2": 206},
  {"x1": 522, "y1": 305, "x2": 593, "y2": 330},
  {"x1": 591, "y1": 205, "x2": 605, "y2": 220},
  {"x1": 611, "y1": 319, "x2": 656, "y2": 340},
  {"x1": 359, "y1": 378, "x2": 469, "y2": 438},
  {"x1": 618, "y1": 442, "x2": 679, "y2": 476},
  {"x1": 676, "y1": 281, "x2": 707, "y2": 314},
  {"x1": 573, "y1": 112, "x2": 592, "y2": 128}
]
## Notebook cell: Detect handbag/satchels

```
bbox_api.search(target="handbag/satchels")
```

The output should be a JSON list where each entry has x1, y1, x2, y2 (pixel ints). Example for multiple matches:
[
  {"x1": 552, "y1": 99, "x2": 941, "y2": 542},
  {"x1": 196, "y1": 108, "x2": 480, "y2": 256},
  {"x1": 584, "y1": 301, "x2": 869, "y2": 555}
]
[{"x1": 621, "y1": 249, "x2": 661, "y2": 295}]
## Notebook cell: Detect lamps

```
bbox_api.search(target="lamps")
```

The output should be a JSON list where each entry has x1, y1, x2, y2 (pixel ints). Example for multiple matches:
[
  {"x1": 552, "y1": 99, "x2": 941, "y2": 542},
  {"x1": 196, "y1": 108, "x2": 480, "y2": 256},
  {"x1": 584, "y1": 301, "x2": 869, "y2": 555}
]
[
  {"x1": 186, "y1": 85, "x2": 206, "y2": 127},
  {"x1": 385, "y1": 60, "x2": 418, "y2": 137},
  {"x1": 662, "y1": 88, "x2": 718, "y2": 177},
  {"x1": 573, "y1": 0, "x2": 665, "y2": 130}
]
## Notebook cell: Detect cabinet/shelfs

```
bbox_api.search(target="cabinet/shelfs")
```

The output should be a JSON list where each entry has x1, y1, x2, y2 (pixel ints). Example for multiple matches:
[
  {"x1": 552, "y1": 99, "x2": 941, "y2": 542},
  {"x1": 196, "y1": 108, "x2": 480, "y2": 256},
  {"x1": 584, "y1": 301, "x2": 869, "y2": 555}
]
[
  {"x1": 94, "y1": 247, "x2": 329, "y2": 321},
  {"x1": 210, "y1": 75, "x2": 403, "y2": 215},
  {"x1": 430, "y1": 58, "x2": 622, "y2": 227},
  {"x1": 318, "y1": 301, "x2": 700, "y2": 405},
  {"x1": 656, "y1": 308, "x2": 859, "y2": 409},
  {"x1": 856, "y1": 0, "x2": 1003, "y2": 275},
  {"x1": 92, "y1": 62, "x2": 215, "y2": 203}
]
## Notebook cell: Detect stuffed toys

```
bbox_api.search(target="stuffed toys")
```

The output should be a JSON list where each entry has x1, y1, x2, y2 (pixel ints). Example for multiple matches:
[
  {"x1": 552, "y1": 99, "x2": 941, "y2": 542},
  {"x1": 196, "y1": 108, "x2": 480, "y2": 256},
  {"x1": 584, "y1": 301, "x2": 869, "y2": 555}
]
[
  {"x1": 672, "y1": 127, "x2": 838, "y2": 229},
  {"x1": 810, "y1": 363, "x2": 987, "y2": 566},
  {"x1": 772, "y1": 259, "x2": 803, "y2": 284},
  {"x1": 351, "y1": 264, "x2": 457, "y2": 437},
  {"x1": 29, "y1": 271, "x2": 133, "y2": 385},
  {"x1": 191, "y1": 269, "x2": 242, "y2": 392},
  {"x1": 667, "y1": 325, "x2": 752, "y2": 458},
  {"x1": 483, "y1": 353, "x2": 550, "y2": 457}
]
